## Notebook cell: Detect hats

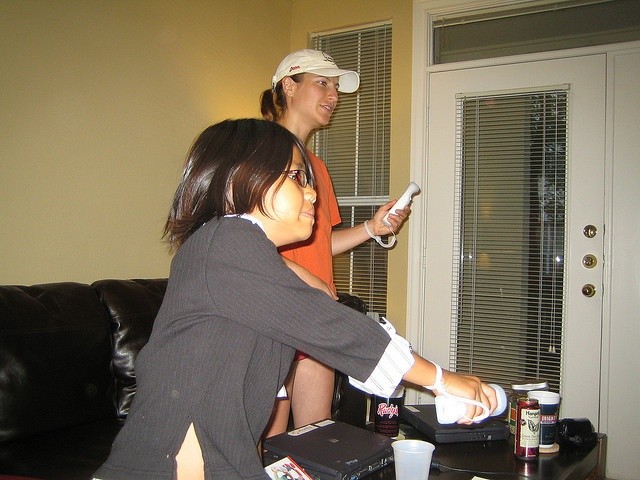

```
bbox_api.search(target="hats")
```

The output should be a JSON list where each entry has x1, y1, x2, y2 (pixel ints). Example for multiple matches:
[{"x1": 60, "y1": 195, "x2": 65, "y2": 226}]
[{"x1": 271, "y1": 48, "x2": 360, "y2": 94}]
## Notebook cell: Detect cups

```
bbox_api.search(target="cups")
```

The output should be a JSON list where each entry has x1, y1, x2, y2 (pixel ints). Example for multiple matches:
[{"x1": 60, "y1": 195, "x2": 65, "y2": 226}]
[
  {"x1": 391, "y1": 440, "x2": 436, "y2": 480},
  {"x1": 373, "y1": 385, "x2": 404, "y2": 437},
  {"x1": 527, "y1": 391, "x2": 561, "y2": 448}
]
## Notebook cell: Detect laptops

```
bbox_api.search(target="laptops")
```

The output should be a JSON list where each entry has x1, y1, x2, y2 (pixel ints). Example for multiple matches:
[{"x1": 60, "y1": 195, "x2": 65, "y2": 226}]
[
  {"x1": 401, "y1": 404, "x2": 510, "y2": 444},
  {"x1": 261, "y1": 418, "x2": 397, "y2": 480}
]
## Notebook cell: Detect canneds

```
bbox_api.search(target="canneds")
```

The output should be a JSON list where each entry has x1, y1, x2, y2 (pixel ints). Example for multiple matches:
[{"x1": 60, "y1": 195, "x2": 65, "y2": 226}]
[
  {"x1": 508, "y1": 380, "x2": 549, "y2": 435},
  {"x1": 516, "y1": 399, "x2": 541, "y2": 462}
]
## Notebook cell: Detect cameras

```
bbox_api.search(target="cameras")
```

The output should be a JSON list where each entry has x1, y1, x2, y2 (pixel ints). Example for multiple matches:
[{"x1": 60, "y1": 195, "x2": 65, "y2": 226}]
[{"x1": 557, "y1": 418, "x2": 597, "y2": 446}]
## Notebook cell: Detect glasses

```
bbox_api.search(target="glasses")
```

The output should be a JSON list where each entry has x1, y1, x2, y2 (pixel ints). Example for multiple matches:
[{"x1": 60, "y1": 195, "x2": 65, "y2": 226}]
[{"x1": 281, "y1": 169, "x2": 314, "y2": 188}]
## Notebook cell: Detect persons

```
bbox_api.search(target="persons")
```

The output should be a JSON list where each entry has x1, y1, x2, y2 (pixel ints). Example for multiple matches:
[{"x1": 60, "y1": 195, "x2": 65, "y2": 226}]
[
  {"x1": 259, "y1": 49, "x2": 411, "y2": 438},
  {"x1": 91, "y1": 117, "x2": 497, "y2": 480}
]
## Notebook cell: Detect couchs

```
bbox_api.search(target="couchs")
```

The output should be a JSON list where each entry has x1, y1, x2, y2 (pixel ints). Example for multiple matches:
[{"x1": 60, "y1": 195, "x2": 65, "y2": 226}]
[{"x1": 0, "y1": 278, "x2": 369, "y2": 480}]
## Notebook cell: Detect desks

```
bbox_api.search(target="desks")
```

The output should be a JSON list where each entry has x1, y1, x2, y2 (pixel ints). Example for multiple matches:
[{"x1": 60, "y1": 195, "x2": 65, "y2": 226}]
[{"x1": 334, "y1": 413, "x2": 607, "y2": 480}]
